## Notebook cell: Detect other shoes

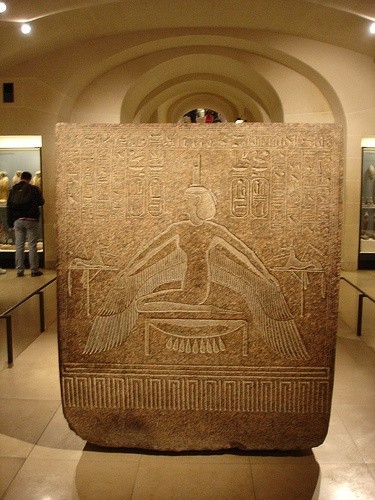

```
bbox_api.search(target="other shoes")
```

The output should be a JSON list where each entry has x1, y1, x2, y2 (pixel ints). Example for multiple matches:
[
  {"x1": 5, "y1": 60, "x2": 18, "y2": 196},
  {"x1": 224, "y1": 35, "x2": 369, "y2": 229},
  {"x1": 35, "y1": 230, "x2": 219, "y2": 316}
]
[
  {"x1": 0, "y1": 268, "x2": 7, "y2": 273},
  {"x1": 31, "y1": 271, "x2": 43, "y2": 277},
  {"x1": 18, "y1": 274, "x2": 24, "y2": 277}
]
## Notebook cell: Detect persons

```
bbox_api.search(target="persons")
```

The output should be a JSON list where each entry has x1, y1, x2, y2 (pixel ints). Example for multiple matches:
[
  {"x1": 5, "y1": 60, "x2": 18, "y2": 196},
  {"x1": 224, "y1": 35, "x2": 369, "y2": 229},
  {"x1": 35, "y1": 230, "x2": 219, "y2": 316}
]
[
  {"x1": 0, "y1": 269, "x2": 6, "y2": 273},
  {"x1": 184, "y1": 109, "x2": 222, "y2": 123},
  {"x1": 6, "y1": 171, "x2": 44, "y2": 277}
]
